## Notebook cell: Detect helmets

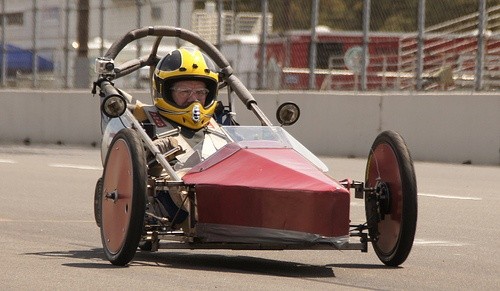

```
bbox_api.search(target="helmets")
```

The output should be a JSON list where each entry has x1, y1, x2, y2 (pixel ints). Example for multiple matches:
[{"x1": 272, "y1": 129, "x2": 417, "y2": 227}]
[{"x1": 152, "y1": 48, "x2": 220, "y2": 130}]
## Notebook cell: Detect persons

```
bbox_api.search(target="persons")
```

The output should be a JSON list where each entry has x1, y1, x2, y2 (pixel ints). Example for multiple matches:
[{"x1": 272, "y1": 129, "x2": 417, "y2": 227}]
[{"x1": 131, "y1": 47, "x2": 242, "y2": 226}]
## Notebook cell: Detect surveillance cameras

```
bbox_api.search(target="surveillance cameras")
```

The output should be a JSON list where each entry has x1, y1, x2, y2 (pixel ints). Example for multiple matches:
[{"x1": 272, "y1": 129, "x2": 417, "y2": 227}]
[{"x1": 94, "y1": 57, "x2": 115, "y2": 78}]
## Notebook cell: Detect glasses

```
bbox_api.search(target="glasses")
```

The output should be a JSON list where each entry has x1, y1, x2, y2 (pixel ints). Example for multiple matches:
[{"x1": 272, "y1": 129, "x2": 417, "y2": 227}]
[{"x1": 168, "y1": 87, "x2": 210, "y2": 99}]
